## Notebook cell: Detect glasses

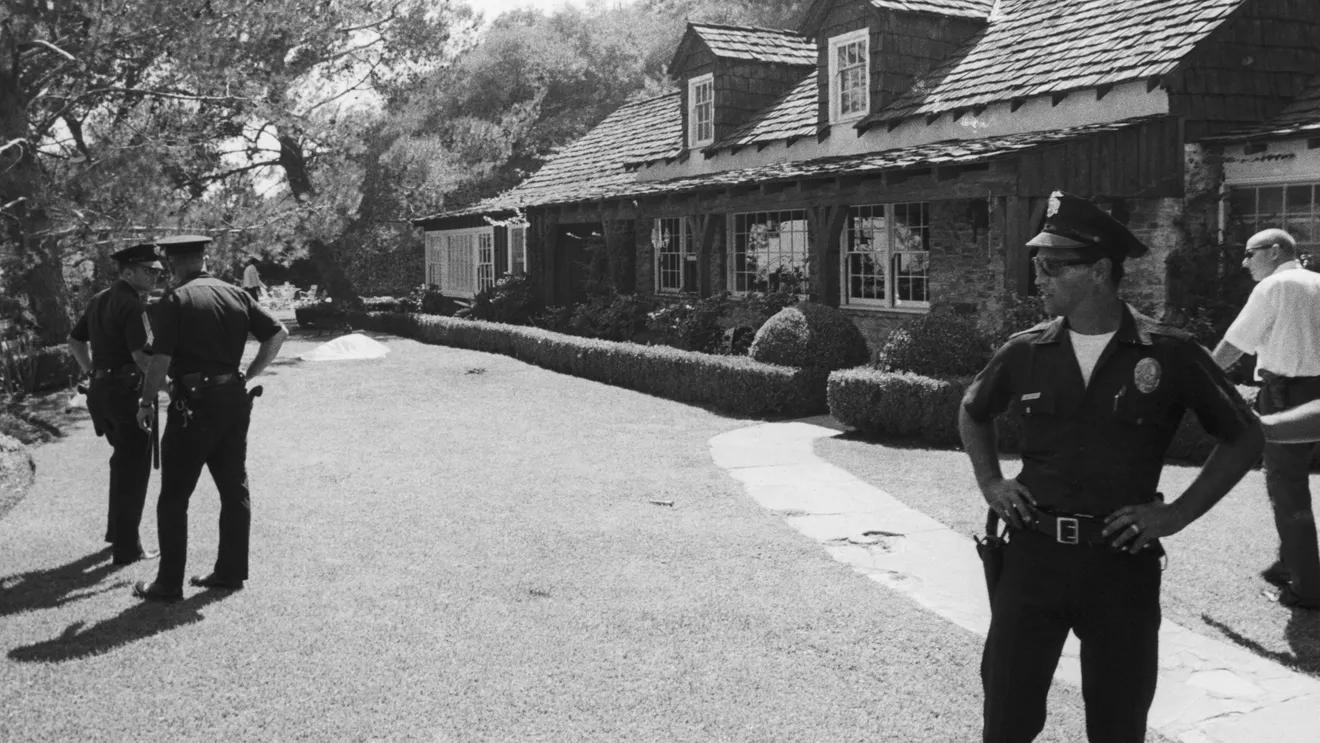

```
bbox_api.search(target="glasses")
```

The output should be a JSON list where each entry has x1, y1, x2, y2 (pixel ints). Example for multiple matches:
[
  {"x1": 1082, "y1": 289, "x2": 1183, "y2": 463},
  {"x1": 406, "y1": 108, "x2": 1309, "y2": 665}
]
[
  {"x1": 1030, "y1": 256, "x2": 1094, "y2": 278},
  {"x1": 1246, "y1": 243, "x2": 1283, "y2": 260}
]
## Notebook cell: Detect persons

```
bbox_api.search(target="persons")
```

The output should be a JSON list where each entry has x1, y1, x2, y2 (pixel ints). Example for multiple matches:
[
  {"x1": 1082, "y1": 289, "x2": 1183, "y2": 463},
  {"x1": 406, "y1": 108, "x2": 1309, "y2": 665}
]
[
  {"x1": 1212, "y1": 229, "x2": 1320, "y2": 610},
  {"x1": 135, "y1": 235, "x2": 290, "y2": 602},
  {"x1": 958, "y1": 194, "x2": 1266, "y2": 743},
  {"x1": 67, "y1": 243, "x2": 172, "y2": 564},
  {"x1": 243, "y1": 258, "x2": 260, "y2": 302}
]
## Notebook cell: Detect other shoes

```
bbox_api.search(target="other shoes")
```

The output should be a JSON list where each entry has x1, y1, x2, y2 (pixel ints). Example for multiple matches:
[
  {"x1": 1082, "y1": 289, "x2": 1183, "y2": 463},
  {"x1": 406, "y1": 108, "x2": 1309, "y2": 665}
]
[
  {"x1": 1261, "y1": 586, "x2": 1320, "y2": 609},
  {"x1": 114, "y1": 548, "x2": 160, "y2": 566},
  {"x1": 134, "y1": 580, "x2": 182, "y2": 602},
  {"x1": 192, "y1": 572, "x2": 243, "y2": 588}
]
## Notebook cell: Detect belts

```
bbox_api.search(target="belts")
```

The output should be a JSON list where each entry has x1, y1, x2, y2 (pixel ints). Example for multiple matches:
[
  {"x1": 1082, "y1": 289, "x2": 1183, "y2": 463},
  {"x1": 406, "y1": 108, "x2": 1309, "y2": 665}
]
[
  {"x1": 1017, "y1": 502, "x2": 1126, "y2": 545},
  {"x1": 198, "y1": 371, "x2": 239, "y2": 390}
]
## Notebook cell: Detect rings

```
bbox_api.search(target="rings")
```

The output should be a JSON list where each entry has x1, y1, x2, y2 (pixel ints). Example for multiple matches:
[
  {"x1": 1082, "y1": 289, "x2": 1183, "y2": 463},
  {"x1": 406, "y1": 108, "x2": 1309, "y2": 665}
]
[{"x1": 1131, "y1": 525, "x2": 1139, "y2": 533}]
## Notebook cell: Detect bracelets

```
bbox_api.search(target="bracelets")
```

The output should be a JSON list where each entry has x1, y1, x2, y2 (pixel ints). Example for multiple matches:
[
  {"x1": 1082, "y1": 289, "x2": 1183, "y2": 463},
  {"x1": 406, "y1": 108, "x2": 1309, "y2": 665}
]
[{"x1": 139, "y1": 398, "x2": 152, "y2": 408}]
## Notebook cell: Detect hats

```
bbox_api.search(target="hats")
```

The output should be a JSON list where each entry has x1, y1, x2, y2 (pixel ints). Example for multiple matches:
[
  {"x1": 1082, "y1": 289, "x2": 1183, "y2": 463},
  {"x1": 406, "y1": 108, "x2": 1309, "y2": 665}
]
[
  {"x1": 110, "y1": 243, "x2": 165, "y2": 272},
  {"x1": 156, "y1": 237, "x2": 213, "y2": 256},
  {"x1": 1025, "y1": 188, "x2": 1149, "y2": 264}
]
